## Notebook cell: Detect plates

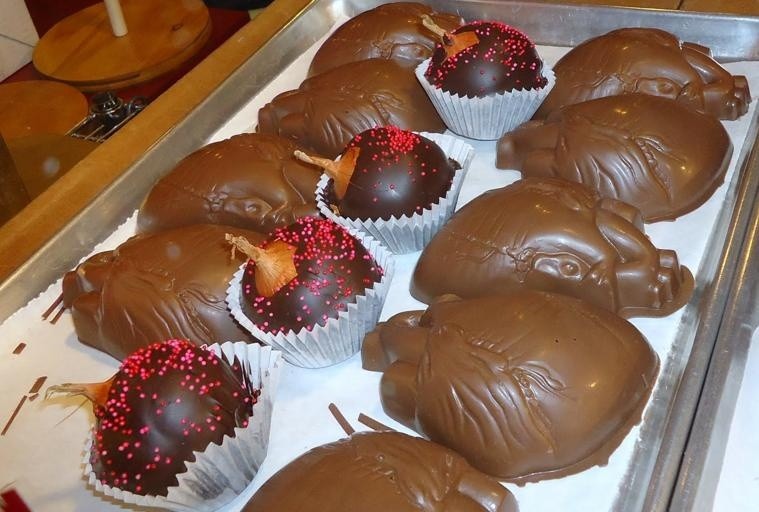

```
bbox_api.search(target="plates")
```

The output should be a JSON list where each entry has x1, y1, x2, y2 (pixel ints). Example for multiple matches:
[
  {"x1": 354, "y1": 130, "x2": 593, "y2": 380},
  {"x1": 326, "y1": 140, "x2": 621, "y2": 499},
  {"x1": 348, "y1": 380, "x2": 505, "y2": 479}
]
[
  {"x1": 668, "y1": 198, "x2": 759, "y2": 512},
  {"x1": 0, "y1": 1, "x2": 759, "y2": 512}
]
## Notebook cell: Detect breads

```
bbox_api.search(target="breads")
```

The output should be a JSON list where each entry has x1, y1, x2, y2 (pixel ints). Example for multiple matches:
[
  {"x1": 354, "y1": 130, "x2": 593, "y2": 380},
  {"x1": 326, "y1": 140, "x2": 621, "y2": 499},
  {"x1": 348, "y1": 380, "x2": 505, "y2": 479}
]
[
  {"x1": 136, "y1": 133, "x2": 332, "y2": 238},
  {"x1": 225, "y1": 216, "x2": 395, "y2": 369},
  {"x1": 295, "y1": 126, "x2": 476, "y2": 255},
  {"x1": 307, "y1": 2, "x2": 462, "y2": 71},
  {"x1": 361, "y1": 289, "x2": 660, "y2": 481},
  {"x1": 62, "y1": 224, "x2": 267, "y2": 364},
  {"x1": 415, "y1": 14, "x2": 557, "y2": 140},
  {"x1": 45, "y1": 339, "x2": 284, "y2": 512},
  {"x1": 256, "y1": 58, "x2": 448, "y2": 157},
  {"x1": 410, "y1": 176, "x2": 694, "y2": 320},
  {"x1": 530, "y1": 28, "x2": 752, "y2": 121},
  {"x1": 241, "y1": 430, "x2": 516, "y2": 512},
  {"x1": 496, "y1": 93, "x2": 734, "y2": 224}
]
